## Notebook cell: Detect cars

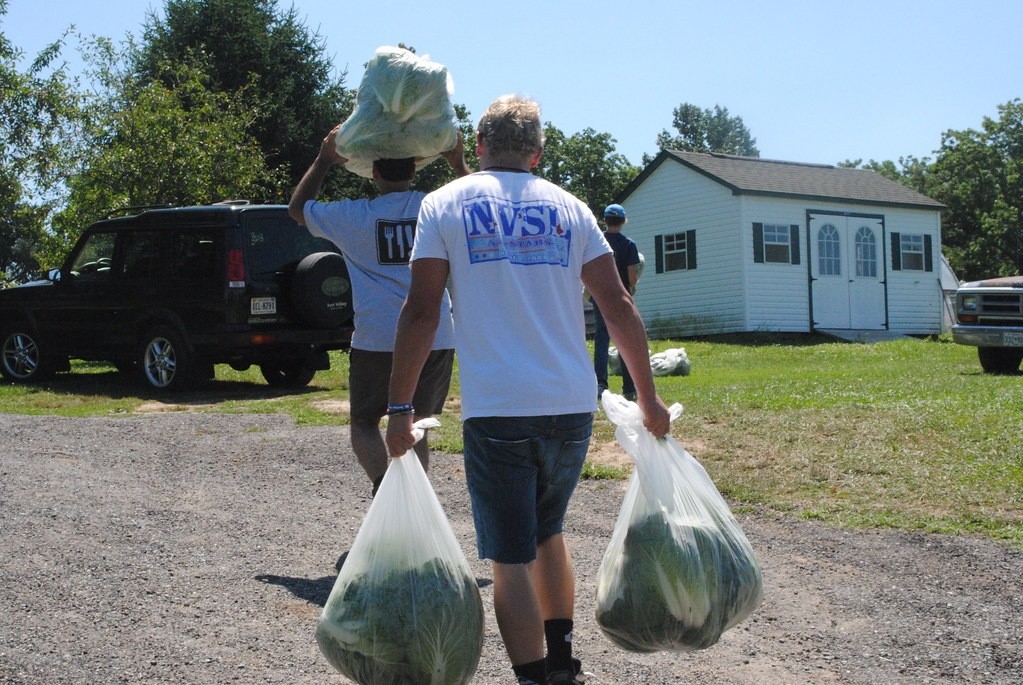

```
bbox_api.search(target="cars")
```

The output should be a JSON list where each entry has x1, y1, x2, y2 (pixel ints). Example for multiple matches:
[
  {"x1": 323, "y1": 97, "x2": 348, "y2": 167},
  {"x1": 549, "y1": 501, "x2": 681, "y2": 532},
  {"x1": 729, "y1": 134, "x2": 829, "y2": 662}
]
[{"x1": 951, "y1": 274, "x2": 1023, "y2": 373}]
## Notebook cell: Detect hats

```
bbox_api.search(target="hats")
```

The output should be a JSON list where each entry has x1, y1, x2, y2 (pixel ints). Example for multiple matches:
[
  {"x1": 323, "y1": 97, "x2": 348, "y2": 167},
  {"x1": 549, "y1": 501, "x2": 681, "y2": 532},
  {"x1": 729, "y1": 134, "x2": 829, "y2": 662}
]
[{"x1": 604, "y1": 204, "x2": 625, "y2": 219}]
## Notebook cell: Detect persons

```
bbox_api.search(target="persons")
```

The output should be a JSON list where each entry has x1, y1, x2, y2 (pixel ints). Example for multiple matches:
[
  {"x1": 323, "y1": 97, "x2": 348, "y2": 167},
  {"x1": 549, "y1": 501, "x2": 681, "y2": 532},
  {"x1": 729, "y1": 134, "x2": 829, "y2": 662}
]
[
  {"x1": 386, "y1": 95, "x2": 670, "y2": 684},
  {"x1": 290, "y1": 119, "x2": 472, "y2": 494},
  {"x1": 582, "y1": 203, "x2": 641, "y2": 403}
]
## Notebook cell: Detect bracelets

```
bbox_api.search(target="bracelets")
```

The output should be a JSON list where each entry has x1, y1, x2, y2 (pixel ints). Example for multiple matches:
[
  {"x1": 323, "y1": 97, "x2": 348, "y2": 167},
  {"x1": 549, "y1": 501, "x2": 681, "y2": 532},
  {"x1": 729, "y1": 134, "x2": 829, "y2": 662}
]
[{"x1": 387, "y1": 403, "x2": 416, "y2": 416}]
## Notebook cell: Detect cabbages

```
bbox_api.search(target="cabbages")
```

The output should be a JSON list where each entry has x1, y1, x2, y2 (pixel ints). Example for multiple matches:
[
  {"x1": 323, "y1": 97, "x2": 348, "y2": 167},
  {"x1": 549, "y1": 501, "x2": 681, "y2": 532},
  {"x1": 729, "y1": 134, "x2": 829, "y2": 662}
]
[
  {"x1": 597, "y1": 512, "x2": 756, "y2": 652},
  {"x1": 315, "y1": 557, "x2": 485, "y2": 685},
  {"x1": 335, "y1": 42, "x2": 457, "y2": 178}
]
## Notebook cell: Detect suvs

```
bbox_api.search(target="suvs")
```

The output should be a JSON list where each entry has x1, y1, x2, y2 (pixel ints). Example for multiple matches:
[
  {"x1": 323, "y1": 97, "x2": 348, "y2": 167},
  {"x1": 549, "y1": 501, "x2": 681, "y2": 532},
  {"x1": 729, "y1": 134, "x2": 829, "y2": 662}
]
[{"x1": 0, "y1": 199, "x2": 354, "y2": 398}]
